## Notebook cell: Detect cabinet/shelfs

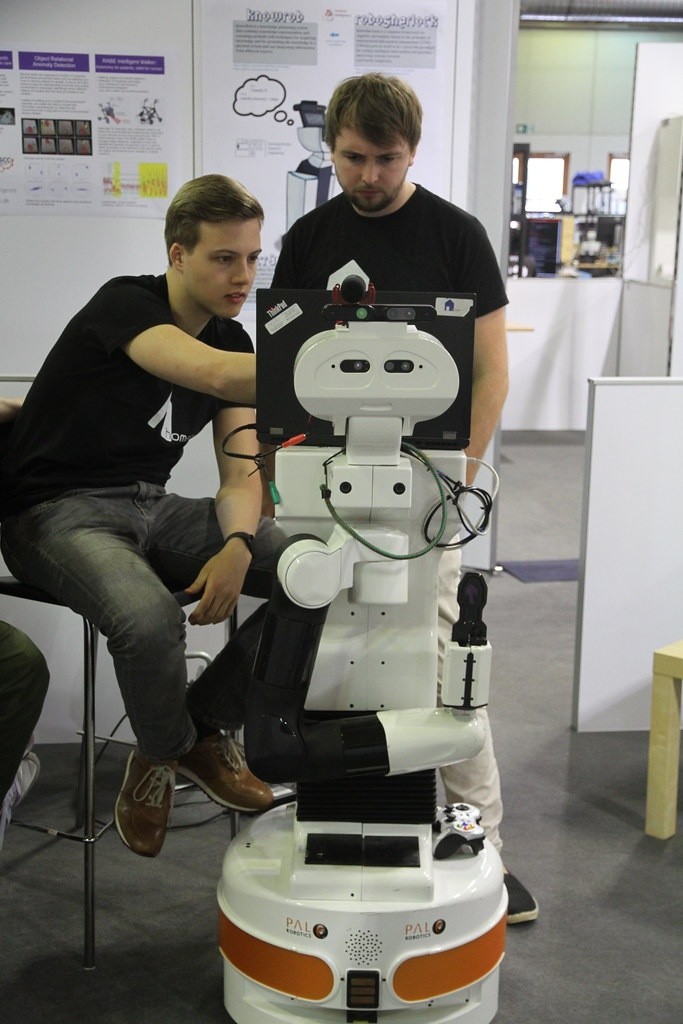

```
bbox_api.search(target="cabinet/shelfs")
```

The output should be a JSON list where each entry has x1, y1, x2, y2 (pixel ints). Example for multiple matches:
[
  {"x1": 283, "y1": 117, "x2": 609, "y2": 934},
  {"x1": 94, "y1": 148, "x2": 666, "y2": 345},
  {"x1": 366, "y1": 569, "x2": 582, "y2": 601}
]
[
  {"x1": 510, "y1": 143, "x2": 624, "y2": 277},
  {"x1": 616, "y1": 42, "x2": 683, "y2": 376}
]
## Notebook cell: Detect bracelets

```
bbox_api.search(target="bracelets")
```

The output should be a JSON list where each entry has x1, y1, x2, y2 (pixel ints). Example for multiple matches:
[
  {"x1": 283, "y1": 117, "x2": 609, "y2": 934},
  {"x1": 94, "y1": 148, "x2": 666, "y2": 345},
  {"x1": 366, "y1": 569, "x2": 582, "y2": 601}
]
[{"x1": 224, "y1": 531, "x2": 257, "y2": 560}]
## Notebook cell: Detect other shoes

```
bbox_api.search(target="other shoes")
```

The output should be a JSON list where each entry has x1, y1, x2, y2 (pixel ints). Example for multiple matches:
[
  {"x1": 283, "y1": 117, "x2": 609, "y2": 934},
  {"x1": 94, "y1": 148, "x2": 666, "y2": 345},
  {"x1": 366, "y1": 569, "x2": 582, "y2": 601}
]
[
  {"x1": 503, "y1": 864, "x2": 540, "y2": 925},
  {"x1": 0, "y1": 751, "x2": 41, "y2": 849}
]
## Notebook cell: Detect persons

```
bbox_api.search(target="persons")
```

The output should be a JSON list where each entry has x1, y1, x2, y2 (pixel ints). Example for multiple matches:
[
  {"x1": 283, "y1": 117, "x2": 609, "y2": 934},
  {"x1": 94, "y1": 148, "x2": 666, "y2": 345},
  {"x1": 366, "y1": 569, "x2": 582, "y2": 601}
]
[
  {"x1": 0, "y1": 174, "x2": 289, "y2": 858},
  {"x1": 268, "y1": 72, "x2": 540, "y2": 927},
  {"x1": 0, "y1": 620, "x2": 51, "y2": 852}
]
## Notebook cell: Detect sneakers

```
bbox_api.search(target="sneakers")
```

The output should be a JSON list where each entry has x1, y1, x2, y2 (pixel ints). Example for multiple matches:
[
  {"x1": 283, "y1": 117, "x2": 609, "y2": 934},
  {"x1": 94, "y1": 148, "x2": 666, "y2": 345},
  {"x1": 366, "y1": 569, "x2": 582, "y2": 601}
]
[
  {"x1": 173, "y1": 726, "x2": 274, "y2": 812},
  {"x1": 114, "y1": 746, "x2": 178, "y2": 857}
]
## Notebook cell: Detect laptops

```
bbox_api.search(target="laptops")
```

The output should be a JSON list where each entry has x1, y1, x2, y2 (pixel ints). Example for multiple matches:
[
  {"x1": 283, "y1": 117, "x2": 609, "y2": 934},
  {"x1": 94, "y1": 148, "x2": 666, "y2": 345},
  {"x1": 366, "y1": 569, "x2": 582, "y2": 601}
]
[{"x1": 252, "y1": 288, "x2": 476, "y2": 450}]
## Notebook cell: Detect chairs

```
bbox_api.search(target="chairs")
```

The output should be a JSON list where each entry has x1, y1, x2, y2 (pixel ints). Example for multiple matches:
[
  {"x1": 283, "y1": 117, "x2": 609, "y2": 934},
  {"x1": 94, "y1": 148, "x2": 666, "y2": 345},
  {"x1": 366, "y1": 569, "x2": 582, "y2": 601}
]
[
  {"x1": 1, "y1": 396, "x2": 239, "y2": 968},
  {"x1": 645, "y1": 637, "x2": 683, "y2": 839}
]
